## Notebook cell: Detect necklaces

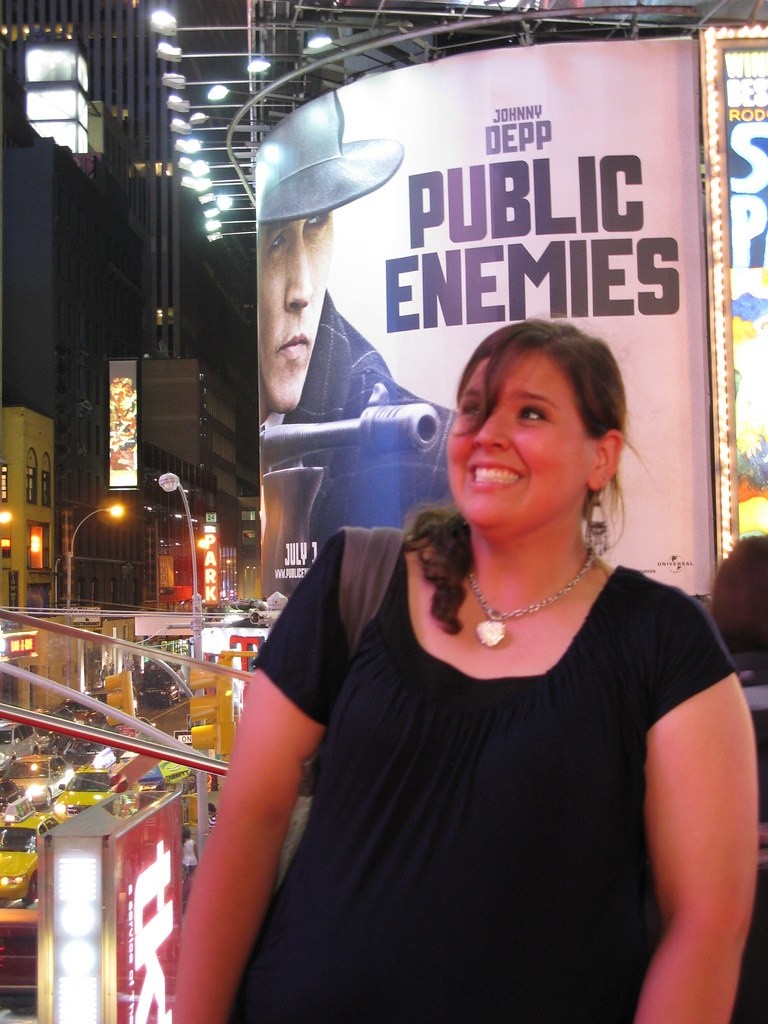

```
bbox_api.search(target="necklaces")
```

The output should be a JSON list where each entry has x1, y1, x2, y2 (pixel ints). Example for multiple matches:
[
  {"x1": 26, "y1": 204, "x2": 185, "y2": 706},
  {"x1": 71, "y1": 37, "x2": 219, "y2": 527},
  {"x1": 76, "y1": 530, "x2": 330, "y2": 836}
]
[{"x1": 465, "y1": 548, "x2": 596, "y2": 648}]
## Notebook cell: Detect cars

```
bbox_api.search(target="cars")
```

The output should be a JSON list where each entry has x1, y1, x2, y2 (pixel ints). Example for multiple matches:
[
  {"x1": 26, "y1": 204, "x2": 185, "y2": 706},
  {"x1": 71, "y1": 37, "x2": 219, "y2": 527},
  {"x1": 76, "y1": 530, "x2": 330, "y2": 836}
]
[
  {"x1": 0, "y1": 797, "x2": 60, "y2": 905},
  {"x1": 54, "y1": 747, "x2": 121, "y2": 820},
  {"x1": 33, "y1": 693, "x2": 107, "y2": 761},
  {"x1": 0, "y1": 775, "x2": 25, "y2": 806},
  {"x1": 0, "y1": 908, "x2": 39, "y2": 1016},
  {"x1": 4, "y1": 753, "x2": 74, "y2": 806},
  {"x1": 142, "y1": 681, "x2": 181, "y2": 706}
]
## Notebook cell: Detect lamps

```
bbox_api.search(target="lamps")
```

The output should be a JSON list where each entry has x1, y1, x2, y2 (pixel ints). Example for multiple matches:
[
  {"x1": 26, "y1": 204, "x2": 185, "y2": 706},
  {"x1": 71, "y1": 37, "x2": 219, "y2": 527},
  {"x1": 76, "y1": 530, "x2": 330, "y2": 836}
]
[{"x1": 149, "y1": 12, "x2": 396, "y2": 244}]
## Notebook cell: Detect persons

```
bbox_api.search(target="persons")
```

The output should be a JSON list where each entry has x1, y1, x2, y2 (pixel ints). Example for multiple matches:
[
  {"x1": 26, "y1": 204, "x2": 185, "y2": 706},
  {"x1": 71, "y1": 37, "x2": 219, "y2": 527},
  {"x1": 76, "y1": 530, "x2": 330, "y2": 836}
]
[
  {"x1": 172, "y1": 319, "x2": 761, "y2": 1024},
  {"x1": 707, "y1": 535, "x2": 768, "y2": 870},
  {"x1": 257, "y1": 92, "x2": 458, "y2": 578}
]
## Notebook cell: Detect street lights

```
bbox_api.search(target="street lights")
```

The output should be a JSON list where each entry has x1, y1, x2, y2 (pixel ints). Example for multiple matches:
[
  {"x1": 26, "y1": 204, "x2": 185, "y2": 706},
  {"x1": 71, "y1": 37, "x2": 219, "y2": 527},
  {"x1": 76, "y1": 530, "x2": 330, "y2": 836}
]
[
  {"x1": 54, "y1": 557, "x2": 62, "y2": 607},
  {"x1": 159, "y1": 473, "x2": 211, "y2": 850},
  {"x1": 65, "y1": 505, "x2": 125, "y2": 690}
]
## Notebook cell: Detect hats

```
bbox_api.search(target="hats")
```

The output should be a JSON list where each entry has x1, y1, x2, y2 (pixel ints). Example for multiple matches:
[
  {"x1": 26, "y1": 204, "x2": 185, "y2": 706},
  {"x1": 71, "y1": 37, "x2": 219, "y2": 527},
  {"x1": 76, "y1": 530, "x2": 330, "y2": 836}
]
[{"x1": 256, "y1": 90, "x2": 405, "y2": 223}]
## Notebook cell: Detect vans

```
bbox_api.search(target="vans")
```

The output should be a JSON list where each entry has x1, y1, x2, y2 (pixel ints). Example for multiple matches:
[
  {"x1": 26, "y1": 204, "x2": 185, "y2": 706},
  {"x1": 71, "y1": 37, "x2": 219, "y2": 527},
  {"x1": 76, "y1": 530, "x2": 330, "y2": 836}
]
[{"x1": 0, "y1": 722, "x2": 39, "y2": 770}]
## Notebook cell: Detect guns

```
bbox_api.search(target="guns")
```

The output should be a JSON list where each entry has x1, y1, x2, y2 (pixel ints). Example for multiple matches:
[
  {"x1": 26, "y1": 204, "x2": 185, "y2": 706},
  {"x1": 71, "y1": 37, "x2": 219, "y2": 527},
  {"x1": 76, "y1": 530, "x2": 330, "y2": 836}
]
[{"x1": 259, "y1": 381, "x2": 444, "y2": 601}]
